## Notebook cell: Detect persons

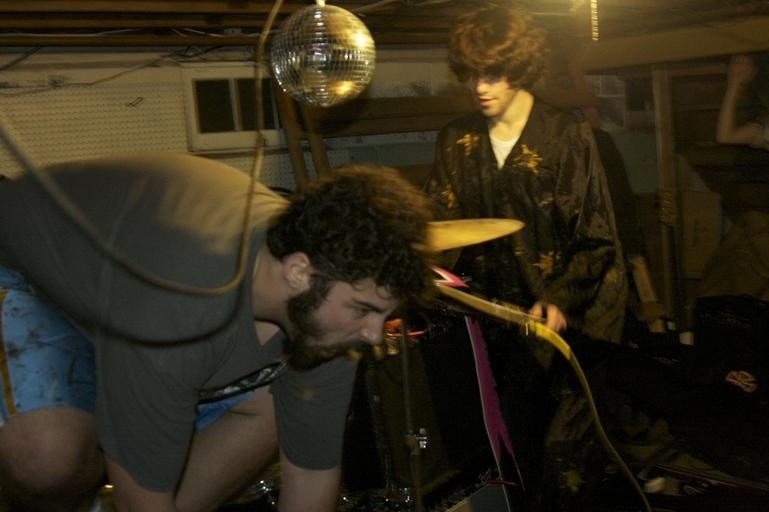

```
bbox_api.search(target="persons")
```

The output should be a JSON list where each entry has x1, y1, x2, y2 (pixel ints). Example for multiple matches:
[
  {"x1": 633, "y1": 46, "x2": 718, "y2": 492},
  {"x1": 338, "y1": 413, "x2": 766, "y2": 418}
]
[
  {"x1": 421, "y1": 3, "x2": 619, "y2": 511},
  {"x1": 0, "y1": 157, "x2": 438, "y2": 511},
  {"x1": 715, "y1": 53, "x2": 767, "y2": 151}
]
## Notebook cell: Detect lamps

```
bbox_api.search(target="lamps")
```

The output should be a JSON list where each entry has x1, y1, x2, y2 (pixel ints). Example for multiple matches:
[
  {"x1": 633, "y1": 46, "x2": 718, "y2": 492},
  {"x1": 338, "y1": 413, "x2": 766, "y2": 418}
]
[{"x1": 267, "y1": 0, "x2": 381, "y2": 108}]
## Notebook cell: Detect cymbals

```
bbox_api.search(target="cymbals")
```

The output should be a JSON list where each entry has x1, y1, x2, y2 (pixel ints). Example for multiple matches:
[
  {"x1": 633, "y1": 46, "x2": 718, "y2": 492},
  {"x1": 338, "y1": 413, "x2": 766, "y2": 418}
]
[{"x1": 426, "y1": 217, "x2": 524, "y2": 250}]
